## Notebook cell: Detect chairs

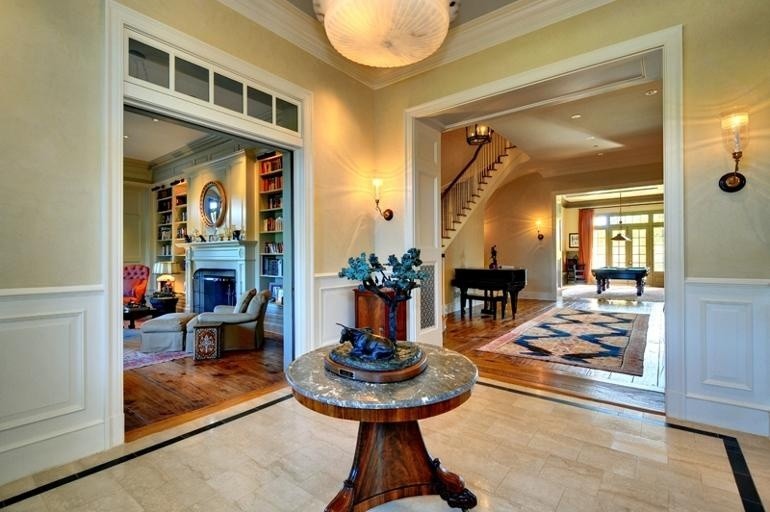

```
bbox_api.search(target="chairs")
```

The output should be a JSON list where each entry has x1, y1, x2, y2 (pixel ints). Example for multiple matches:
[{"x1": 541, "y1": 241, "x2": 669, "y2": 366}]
[{"x1": 123, "y1": 262, "x2": 150, "y2": 330}]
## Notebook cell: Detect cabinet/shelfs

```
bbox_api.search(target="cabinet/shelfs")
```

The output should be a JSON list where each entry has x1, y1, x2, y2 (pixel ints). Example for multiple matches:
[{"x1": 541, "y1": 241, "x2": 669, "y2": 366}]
[
  {"x1": 254, "y1": 148, "x2": 283, "y2": 342},
  {"x1": 566, "y1": 259, "x2": 586, "y2": 285},
  {"x1": 150, "y1": 176, "x2": 189, "y2": 310},
  {"x1": 353, "y1": 283, "x2": 409, "y2": 342},
  {"x1": 174, "y1": 240, "x2": 258, "y2": 313}
]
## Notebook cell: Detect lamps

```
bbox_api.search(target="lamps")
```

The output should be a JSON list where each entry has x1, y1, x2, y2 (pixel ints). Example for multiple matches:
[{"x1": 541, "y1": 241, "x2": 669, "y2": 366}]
[
  {"x1": 153, "y1": 275, "x2": 175, "y2": 298},
  {"x1": 372, "y1": 178, "x2": 393, "y2": 221},
  {"x1": 465, "y1": 123, "x2": 494, "y2": 146},
  {"x1": 534, "y1": 218, "x2": 544, "y2": 240},
  {"x1": 323, "y1": 20, "x2": 451, "y2": 69},
  {"x1": 610, "y1": 191, "x2": 631, "y2": 241},
  {"x1": 718, "y1": 111, "x2": 750, "y2": 193}
]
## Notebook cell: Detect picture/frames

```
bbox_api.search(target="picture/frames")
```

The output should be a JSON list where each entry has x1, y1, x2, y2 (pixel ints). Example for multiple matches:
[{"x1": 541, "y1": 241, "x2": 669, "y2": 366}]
[
  {"x1": 569, "y1": 233, "x2": 580, "y2": 248},
  {"x1": 269, "y1": 281, "x2": 283, "y2": 299}
]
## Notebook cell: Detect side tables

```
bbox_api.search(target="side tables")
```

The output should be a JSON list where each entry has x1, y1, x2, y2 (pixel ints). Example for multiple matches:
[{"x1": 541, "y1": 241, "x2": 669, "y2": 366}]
[{"x1": 150, "y1": 295, "x2": 178, "y2": 316}]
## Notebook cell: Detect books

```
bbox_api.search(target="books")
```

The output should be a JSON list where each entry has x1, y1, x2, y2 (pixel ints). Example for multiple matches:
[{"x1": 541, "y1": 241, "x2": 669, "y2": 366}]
[
  {"x1": 264, "y1": 258, "x2": 282, "y2": 276},
  {"x1": 264, "y1": 242, "x2": 283, "y2": 253},
  {"x1": 263, "y1": 175, "x2": 283, "y2": 191},
  {"x1": 261, "y1": 158, "x2": 282, "y2": 173},
  {"x1": 268, "y1": 282, "x2": 283, "y2": 306},
  {"x1": 269, "y1": 197, "x2": 282, "y2": 209},
  {"x1": 263, "y1": 217, "x2": 283, "y2": 231}
]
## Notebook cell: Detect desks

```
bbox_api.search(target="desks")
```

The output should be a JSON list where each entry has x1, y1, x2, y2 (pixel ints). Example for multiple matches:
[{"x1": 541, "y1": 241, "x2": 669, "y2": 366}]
[{"x1": 286, "y1": 340, "x2": 479, "y2": 512}]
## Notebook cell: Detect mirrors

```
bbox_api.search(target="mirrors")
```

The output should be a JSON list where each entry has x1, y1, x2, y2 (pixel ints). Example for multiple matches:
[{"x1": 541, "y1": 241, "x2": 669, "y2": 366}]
[{"x1": 199, "y1": 180, "x2": 227, "y2": 227}]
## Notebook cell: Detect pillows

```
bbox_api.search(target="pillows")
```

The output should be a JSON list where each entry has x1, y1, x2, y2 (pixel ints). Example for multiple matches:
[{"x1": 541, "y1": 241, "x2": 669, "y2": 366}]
[{"x1": 123, "y1": 279, "x2": 144, "y2": 297}]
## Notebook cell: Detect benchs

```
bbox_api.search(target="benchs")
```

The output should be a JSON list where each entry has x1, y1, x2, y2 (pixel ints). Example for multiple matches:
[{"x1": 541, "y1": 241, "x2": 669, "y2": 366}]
[{"x1": 137, "y1": 313, "x2": 199, "y2": 354}]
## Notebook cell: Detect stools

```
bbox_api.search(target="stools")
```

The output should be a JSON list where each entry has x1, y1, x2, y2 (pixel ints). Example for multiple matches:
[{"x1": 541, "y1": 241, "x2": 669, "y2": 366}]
[{"x1": 462, "y1": 293, "x2": 505, "y2": 321}]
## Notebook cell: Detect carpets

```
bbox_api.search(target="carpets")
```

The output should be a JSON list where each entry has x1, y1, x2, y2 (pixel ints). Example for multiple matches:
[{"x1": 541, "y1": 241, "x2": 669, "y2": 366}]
[
  {"x1": 123, "y1": 314, "x2": 196, "y2": 370},
  {"x1": 475, "y1": 305, "x2": 650, "y2": 376}
]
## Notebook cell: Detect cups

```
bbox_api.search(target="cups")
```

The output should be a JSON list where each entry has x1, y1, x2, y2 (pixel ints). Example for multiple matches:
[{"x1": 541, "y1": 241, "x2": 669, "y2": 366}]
[
  {"x1": 226, "y1": 232, "x2": 233, "y2": 242},
  {"x1": 219, "y1": 234, "x2": 225, "y2": 241}
]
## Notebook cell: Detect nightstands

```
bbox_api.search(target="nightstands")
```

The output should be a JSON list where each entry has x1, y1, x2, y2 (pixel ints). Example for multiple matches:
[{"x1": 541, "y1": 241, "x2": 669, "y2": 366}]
[{"x1": 193, "y1": 321, "x2": 224, "y2": 363}]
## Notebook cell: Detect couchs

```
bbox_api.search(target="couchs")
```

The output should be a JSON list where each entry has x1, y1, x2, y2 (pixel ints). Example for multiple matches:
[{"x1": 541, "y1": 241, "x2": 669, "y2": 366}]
[
  {"x1": 213, "y1": 289, "x2": 256, "y2": 317},
  {"x1": 185, "y1": 290, "x2": 271, "y2": 355}
]
